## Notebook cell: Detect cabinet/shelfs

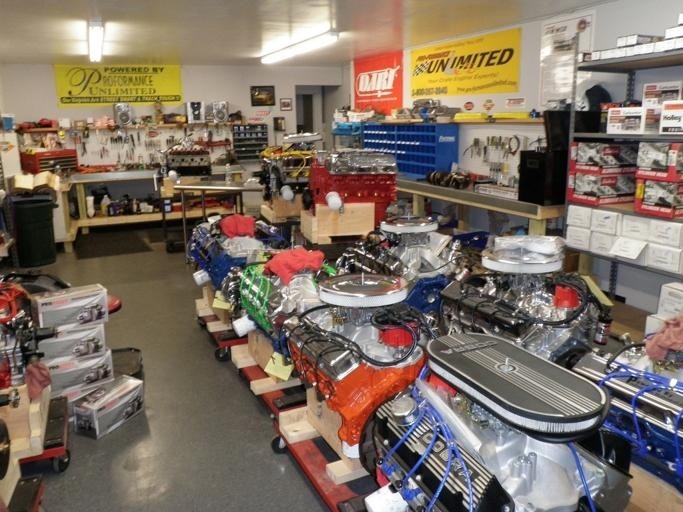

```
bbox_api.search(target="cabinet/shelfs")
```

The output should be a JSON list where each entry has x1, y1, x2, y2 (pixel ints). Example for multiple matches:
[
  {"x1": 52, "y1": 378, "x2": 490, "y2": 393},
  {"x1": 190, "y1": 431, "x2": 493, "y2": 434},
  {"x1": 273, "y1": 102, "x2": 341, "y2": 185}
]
[
  {"x1": 564, "y1": 53, "x2": 683, "y2": 271},
  {"x1": 392, "y1": 182, "x2": 565, "y2": 264},
  {"x1": 53, "y1": 168, "x2": 255, "y2": 250}
]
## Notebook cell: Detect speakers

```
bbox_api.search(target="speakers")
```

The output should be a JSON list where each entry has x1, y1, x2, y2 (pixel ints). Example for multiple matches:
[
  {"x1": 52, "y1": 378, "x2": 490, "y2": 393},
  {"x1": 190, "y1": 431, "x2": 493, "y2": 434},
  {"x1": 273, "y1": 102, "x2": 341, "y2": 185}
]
[
  {"x1": 114, "y1": 103, "x2": 129, "y2": 124},
  {"x1": 15, "y1": 200, "x2": 58, "y2": 268},
  {"x1": 517, "y1": 150, "x2": 567, "y2": 207},
  {"x1": 212, "y1": 101, "x2": 228, "y2": 122},
  {"x1": 186, "y1": 100, "x2": 205, "y2": 124}
]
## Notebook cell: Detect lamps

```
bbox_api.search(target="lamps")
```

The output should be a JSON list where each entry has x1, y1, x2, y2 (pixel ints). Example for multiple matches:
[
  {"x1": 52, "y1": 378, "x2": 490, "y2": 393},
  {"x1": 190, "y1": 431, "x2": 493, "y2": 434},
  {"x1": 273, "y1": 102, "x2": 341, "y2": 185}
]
[
  {"x1": 89, "y1": 27, "x2": 103, "y2": 63},
  {"x1": 253, "y1": 30, "x2": 345, "y2": 65}
]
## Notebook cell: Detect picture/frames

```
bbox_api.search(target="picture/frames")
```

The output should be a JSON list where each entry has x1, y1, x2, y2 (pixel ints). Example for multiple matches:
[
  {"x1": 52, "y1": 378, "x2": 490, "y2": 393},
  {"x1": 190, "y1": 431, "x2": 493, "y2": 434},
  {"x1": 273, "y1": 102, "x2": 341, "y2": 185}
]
[
  {"x1": 250, "y1": 86, "x2": 275, "y2": 106},
  {"x1": 280, "y1": 98, "x2": 292, "y2": 111}
]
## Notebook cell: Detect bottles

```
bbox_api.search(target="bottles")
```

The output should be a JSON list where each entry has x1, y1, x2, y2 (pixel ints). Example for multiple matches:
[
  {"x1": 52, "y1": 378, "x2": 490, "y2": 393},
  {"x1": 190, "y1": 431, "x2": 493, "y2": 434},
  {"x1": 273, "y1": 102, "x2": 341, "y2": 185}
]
[
  {"x1": 225, "y1": 164, "x2": 232, "y2": 184},
  {"x1": 594, "y1": 314, "x2": 613, "y2": 346},
  {"x1": 101, "y1": 195, "x2": 111, "y2": 215}
]
